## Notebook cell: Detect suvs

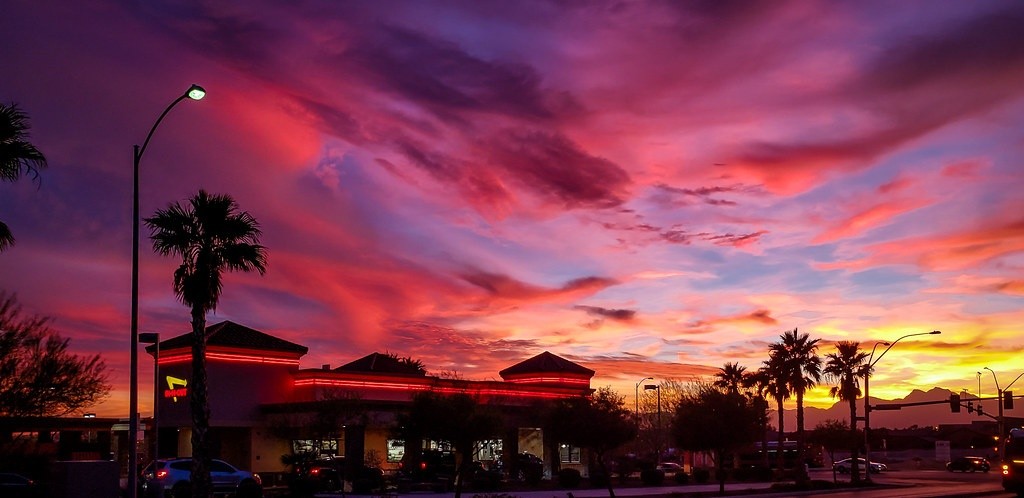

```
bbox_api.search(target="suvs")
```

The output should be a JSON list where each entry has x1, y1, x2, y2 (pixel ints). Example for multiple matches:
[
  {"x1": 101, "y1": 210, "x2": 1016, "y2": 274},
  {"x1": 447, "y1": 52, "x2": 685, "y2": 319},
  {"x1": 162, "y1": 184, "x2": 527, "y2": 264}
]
[
  {"x1": 140, "y1": 456, "x2": 264, "y2": 498},
  {"x1": 282, "y1": 454, "x2": 385, "y2": 498}
]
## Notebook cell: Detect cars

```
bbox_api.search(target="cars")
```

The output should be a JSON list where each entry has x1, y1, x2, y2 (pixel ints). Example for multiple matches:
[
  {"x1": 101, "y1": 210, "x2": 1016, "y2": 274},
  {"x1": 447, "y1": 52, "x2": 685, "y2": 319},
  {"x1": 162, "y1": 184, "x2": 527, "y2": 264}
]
[
  {"x1": 832, "y1": 457, "x2": 888, "y2": 475},
  {"x1": 659, "y1": 462, "x2": 682, "y2": 473},
  {"x1": 393, "y1": 449, "x2": 491, "y2": 493},
  {"x1": 944, "y1": 456, "x2": 991, "y2": 473},
  {"x1": 482, "y1": 452, "x2": 544, "y2": 491}
]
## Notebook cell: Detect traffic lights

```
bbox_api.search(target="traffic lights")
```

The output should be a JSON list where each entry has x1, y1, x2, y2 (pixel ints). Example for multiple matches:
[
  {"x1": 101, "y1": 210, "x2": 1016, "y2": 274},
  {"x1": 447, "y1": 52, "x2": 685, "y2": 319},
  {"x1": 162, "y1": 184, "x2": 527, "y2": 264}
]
[
  {"x1": 978, "y1": 404, "x2": 983, "y2": 416},
  {"x1": 968, "y1": 401, "x2": 973, "y2": 413}
]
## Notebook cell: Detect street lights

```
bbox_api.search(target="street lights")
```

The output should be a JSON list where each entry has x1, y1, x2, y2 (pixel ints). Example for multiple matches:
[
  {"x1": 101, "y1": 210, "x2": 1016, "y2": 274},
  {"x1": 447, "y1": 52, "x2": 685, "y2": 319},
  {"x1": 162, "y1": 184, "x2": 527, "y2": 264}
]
[
  {"x1": 983, "y1": 366, "x2": 1024, "y2": 465},
  {"x1": 126, "y1": 82, "x2": 207, "y2": 498},
  {"x1": 635, "y1": 376, "x2": 653, "y2": 473},
  {"x1": 644, "y1": 384, "x2": 662, "y2": 451},
  {"x1": 139, "y1": 332, "x2": 160, "y2": 479},
  {"x1": 863, "y1": 330, "x2": 941, "y2": 485}
]
